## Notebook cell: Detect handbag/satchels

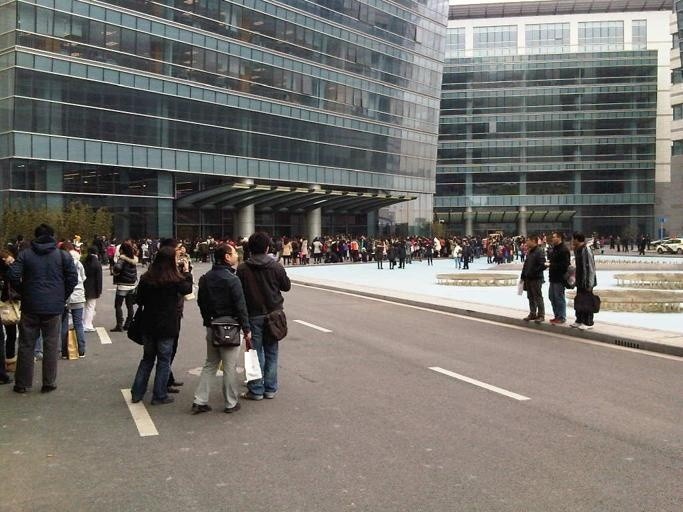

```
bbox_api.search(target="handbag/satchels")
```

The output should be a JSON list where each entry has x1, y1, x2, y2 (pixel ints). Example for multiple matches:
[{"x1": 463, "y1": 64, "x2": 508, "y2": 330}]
[
  {"x1": 562, "y1": 265, "x2": 578, "y2": 289},
  {"x1": 574, "y1": 292, "x2": 601, "y2": 313},
  {"x1": 211, "y1": 316, "x2": 240, "y2": 348},
  {"x1": 265, "y1": 309, "x2": 287, "y2": 344},
  {"x1": 67, "y1": 327, "x2": 80, "y2": 360},
  {"x1": 127, "y1": 312, "x2": 145, "y2": 345},
  {"x1": 0, "y1": 299, "x2": 21, "y2": 324}
]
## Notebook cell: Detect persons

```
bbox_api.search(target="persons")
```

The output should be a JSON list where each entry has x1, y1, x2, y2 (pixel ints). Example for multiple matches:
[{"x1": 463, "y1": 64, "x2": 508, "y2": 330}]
[
  {"x1": 593, "y1": 232, "x2": 650, "y2": 255},
  {"x1": 269, "y1": 232, "x2": 547, "y2": 324},
  {"x1": 81, "y1": 246, "x2": 102, "y2": 333},
  {"x1": 546, "y1": 231, "x2": 570, "y2": 324},
  {"x1": 570, "y1": 234, "x2": 598, "y2": 330},
  {"x1": 159, "y1": 237, "x2": 184, "y2": 394},
  {"x1": 109, "y1": 242, "x2": 139, "y2": 332},
  {"x1": 0, "y1": 223, "x2": 88, "y2": 393},
  {"x1": 177, "y1": 235, "x2": 249, "y2": 274},
  {"x1": 236, "y1": 230, "x2": 291, "y2": 401},
  {"x1": 191, "y1": 242, "x2": 252, "y2": 414},
  {"x1": 93, "y1": 233, "x2": 160, "y2": 276},
  {"x1": 130, "y1": 247, "x2": 193, "y2": 405}
]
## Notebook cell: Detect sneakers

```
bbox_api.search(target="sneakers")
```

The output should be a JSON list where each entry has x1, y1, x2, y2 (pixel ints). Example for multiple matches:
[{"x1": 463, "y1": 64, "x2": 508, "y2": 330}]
[
  {"x1": 242, "y1": 379, "x2": 277, "y2": 401},
  {"x1": 523, "y1": 313, "x2": 594, "y2": 330},
  {"x1": 191, "y1": 402, "x2": 209, "y2": 414},
  {"x1": 225, "y1": 403, "x2": 240, "y2": 413},
  {"x1": 41, "y1": 383, "x2": 57, "y2": 393},
  {"x1": 131, "y1": 380, "x2": 182, "y2": 406},
  {"x1": 13, "y1": 385, "x2": 25, "y2": 393}
]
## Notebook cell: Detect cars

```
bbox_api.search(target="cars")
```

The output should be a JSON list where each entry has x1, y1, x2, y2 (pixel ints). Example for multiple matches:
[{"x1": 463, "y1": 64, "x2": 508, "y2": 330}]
[
  {"x1": 656, "y1": 238, "x2": 683, "y2": 255},
  {"x1": 650, "y1": 237, "x2": 671, "y2": 246}
]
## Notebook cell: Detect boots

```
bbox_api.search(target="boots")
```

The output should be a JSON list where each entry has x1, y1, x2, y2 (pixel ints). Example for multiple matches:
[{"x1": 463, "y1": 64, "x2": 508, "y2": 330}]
[
  {"x1": 5, "y1": 355, "x2": 17, "y2": 372},
  {"x1": 110, "y1": 318, "x2": 123, "y2": 332},
  {"x1": 123, "y1": 316, "x2": 133, "y2": 331}
]
